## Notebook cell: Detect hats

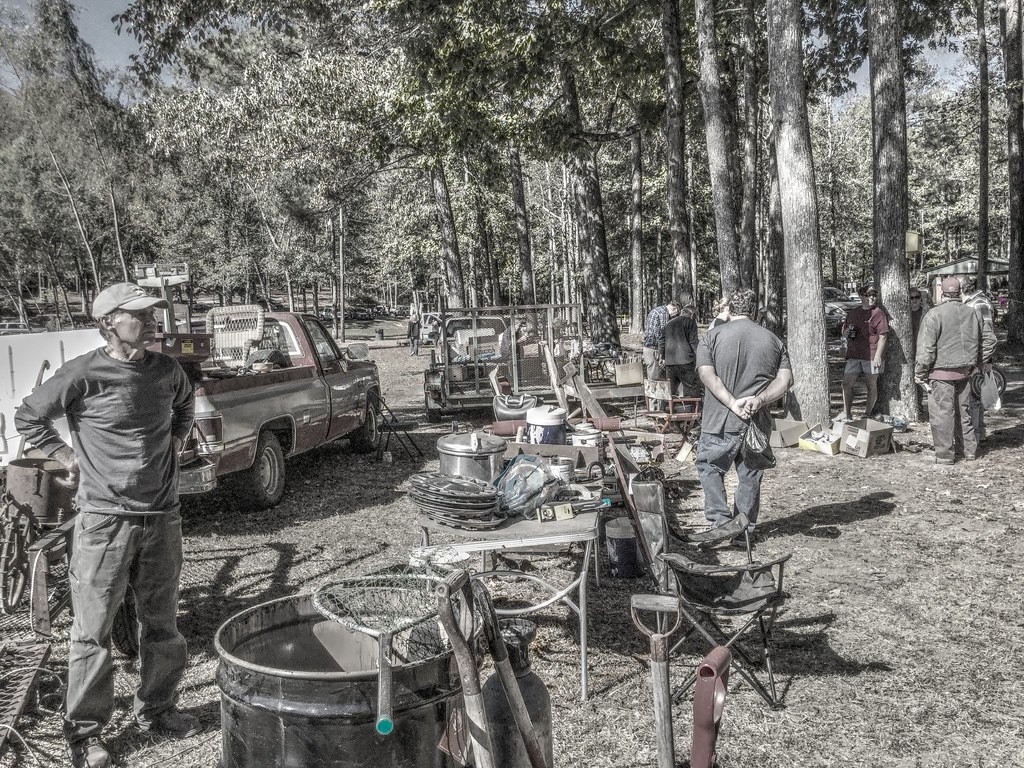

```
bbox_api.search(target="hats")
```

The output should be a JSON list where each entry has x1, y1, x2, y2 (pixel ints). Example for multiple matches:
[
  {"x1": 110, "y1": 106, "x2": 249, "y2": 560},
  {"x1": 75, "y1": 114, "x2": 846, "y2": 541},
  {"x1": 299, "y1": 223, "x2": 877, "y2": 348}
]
[
  {"x1": 859, "y1": 285, "x2": 878, "y2": 295},
  {"x1": 941, "y1": 277, "x2": 961, "y2": 293},
  {"x1": 91, "y1": 282, "x2": 171, "y2": 321}
]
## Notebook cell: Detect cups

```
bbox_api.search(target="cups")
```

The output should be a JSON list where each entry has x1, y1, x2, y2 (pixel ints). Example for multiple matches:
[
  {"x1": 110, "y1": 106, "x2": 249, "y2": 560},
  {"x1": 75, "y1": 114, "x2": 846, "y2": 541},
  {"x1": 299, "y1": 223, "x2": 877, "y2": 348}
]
[{"x1": 848, "y1": 324, "x2": 856, "y2": 337}]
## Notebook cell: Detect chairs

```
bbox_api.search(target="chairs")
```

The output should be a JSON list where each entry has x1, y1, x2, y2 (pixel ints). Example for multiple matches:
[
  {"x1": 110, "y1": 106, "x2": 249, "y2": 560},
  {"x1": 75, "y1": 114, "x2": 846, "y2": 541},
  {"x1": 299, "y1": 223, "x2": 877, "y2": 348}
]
[
  {"x1": 643, "y1": 376, "x2": 702, "y2": 450},
  {"x1": 368, "y1": 390, "x2": 425, "y2": 464},
  {"x1": 544, "y1": 344, "x2": 793, "y2": 709}
]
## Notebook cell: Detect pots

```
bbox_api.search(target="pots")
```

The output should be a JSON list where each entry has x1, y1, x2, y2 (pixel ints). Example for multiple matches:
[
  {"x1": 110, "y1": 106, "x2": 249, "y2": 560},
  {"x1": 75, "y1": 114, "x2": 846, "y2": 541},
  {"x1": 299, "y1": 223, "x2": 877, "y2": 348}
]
[{"x1": 436, "y1": 425, "x2": 507, "y2": 484}]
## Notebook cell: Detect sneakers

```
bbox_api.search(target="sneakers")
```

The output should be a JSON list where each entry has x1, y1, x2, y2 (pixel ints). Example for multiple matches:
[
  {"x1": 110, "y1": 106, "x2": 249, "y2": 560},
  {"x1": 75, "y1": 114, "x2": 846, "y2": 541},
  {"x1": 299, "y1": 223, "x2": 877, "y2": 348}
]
[
  {"x1": 71, "y1": 735, "x2": 111, "y2": 768},
  {"x1": 139, "y1": 706, "x2": 201, "y2": 737}
]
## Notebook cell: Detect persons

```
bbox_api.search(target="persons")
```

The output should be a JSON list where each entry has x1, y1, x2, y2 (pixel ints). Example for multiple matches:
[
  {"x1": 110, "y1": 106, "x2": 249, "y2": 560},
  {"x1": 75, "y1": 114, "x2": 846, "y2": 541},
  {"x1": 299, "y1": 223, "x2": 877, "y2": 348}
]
[
  {"x1": 13, "y1": 282, "x2": 202, "y2": 768},
  {"x1": 911, "y1": 276, "x2": 997, "y2": 464},
  {"x1": 708, "y1": 298, "x2": 731, "y2": 330},
  {"x1": 407, "y1": 315, "x2": 422, "y2": 356},
  {"x1": 696, "y1": 288, "x2": 793, "y2": 546},
  {"x1": 642, "y1": 300, "x2": 704, "y2": 417},
  {"x1": 837, "y1": 286, "x2": 889, "y2": 422}
]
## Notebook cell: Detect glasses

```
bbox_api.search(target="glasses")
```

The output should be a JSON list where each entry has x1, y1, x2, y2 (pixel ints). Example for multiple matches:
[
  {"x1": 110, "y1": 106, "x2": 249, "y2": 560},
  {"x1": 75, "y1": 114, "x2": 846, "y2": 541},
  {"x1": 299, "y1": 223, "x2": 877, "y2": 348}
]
[
  {"x1": 910, "y1": 296, "x2": 920, "y2": 300},
  {"x1": 864, "y1": 293, "x2": 877, "y2": 298}
]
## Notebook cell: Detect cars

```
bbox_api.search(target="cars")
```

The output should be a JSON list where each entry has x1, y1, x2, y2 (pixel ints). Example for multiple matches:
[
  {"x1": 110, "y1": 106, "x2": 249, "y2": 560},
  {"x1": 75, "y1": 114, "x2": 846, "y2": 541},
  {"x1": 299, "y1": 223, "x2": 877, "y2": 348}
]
[
  {"x1": 758, "y1": 285, "x2": 862, "y2": 337},
  {"x1": 320, "y1": 302, "x2": 412, "y2": 322},
  {"x1": 420, "y1": 312, "x2": 454, "y2": 345}
]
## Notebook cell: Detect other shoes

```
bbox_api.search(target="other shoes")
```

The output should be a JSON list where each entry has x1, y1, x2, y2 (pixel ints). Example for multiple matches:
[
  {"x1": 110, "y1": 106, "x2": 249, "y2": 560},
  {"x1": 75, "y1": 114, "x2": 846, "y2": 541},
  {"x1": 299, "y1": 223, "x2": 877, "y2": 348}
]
[
  {"x1": 964, "y1": 454, "x2": 976, "y2": 461},
  {"x1": 936, "y1": 455, "x2": 955, "y2": 465},
  {"x1": 410, "y1": 352, "x2": 415, "y2": 356},
  {"x1": 732, "y1": 532, "x2": 755, "y2": 548}
]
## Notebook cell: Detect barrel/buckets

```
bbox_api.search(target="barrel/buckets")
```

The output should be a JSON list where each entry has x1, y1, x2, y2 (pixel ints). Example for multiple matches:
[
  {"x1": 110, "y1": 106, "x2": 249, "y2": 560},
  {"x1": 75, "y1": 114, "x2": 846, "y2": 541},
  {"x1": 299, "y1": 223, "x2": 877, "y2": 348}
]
[
  {"x1": 409, "y1": 546, "x2": 470, "y2": 573},
  {"x1": 572, "y1": 429, "x2": 604, "y2": 468},
  {"x1": 253, "y1": 351, "x2": 277, "y2": 372},
  {"x1": 606, "y1": 516, "x2": 647, "y2": 578},
  {"x1": 215, "y1": 594, "x2": 483, "y2": 767},
  {"x1": 545, "y1": 455, "x2": 575, "y2": 485},
  {"x1": 7, "y1": 459, "x2": 67, "y2": 526},
  {"x1": 526, "y1": 405, "x2": 567, "y2": 445}
]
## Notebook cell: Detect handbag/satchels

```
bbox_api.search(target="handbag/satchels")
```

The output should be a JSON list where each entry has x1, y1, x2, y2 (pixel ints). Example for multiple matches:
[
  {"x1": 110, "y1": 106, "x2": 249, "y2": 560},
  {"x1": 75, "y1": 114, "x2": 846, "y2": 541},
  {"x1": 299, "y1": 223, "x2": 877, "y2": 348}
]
[
  {"x1": 742, "y1": 412, "x2": 776, "y2": 471},
  {"x1": 981, "y1": 369, "x2": 1001, "y2": 413},
  {"x1": 492, "y1": 393, "x2": 542, "y2": 422}
]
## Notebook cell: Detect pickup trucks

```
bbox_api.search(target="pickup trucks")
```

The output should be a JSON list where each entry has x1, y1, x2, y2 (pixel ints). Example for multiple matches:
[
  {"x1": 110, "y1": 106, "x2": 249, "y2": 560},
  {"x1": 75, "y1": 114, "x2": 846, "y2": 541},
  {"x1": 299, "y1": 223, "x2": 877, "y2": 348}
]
[
  {"x1": 131, "y1": 310, "x2": 386, "y2": 515},
  {"x1": 421, "y1": 314, "x2": 523, "y2": 426}
]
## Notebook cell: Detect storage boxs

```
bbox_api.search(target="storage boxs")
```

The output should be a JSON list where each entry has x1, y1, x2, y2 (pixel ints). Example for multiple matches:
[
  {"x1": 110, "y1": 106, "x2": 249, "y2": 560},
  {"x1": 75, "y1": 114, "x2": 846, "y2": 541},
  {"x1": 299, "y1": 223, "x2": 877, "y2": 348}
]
[{"x1": 768, "y1": 417, "x2": 893, "y2": 457}]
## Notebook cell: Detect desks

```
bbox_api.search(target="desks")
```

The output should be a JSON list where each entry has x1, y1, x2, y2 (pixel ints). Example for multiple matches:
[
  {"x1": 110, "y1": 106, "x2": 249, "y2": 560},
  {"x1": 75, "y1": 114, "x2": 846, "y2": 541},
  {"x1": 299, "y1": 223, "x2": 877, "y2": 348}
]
[{"x1": 410, "y1": 459, "x2": 604, "y2": 700}]
